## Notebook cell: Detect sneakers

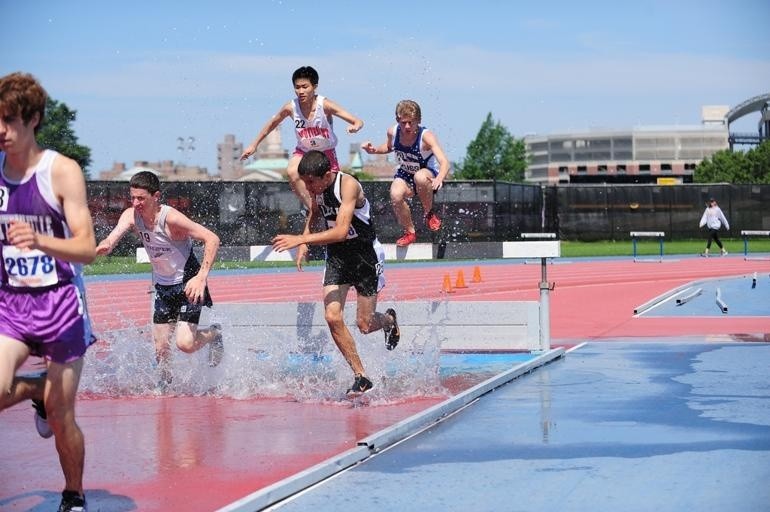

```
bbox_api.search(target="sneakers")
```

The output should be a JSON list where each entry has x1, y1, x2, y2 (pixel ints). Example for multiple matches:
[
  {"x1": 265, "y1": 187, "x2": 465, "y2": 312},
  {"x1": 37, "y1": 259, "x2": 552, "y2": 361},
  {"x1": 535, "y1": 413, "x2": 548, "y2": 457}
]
[
  {"x1": 382, "y1": 307, "x2": 401, "y2": 351},
  {"x1": 32, "y1": 367, "x2": 56, "y2": 441},
  {"x1": 53, "y1": 488, "x2": 89, "y2": 512},
  {"x1": 700, "y1": 252, "x2": 729, "y2": 257},
  {"x1": 206, "y1": 322, "x2": 224, "y2": 368},
  {"x1": 345, "y1": 377, "x2": 376, "y2": 397},
  {"x1": 424, "y1": 214, "x2": 443, "y2": 232},
  {"x1": 394, "y1": 230, "x2": 417, "y2": 247}
]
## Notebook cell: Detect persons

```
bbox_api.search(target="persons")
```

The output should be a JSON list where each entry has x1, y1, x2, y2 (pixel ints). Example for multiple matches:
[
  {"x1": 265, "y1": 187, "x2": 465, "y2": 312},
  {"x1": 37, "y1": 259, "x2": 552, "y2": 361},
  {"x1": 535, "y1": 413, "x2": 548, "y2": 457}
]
[
  {"x1": 239, "y1": 64, "x2": 365, "y2": 213},
  {"x1": 698, "y1": 197, "x2": 730, "y2": 258},
  {"x1": 359, "y1": 100, "x2": 449, "y2": 248},
  {"x1": 0, "y1": 70, "x2": 96, "y2": 511},
  {"x1": 96, "y1": 170, "x2": 227, "y2": 397},
  {"x1": 269, "y1": 151, "x2": 400, "y2": 397}
]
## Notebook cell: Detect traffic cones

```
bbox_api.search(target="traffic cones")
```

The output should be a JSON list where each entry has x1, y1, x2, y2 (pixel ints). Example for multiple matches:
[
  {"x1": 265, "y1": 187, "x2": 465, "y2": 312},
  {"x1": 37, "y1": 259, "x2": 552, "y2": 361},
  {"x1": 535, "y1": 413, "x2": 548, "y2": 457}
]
[
  {"x1": 470, "y1": 266, "x2": 485, "y2": 283},
  {"x1": 455, "y1": 271, "x2": 468, "y2": 288},
  {"x1": 439, "y1": 273, "x2": 456, "y2": 293}
]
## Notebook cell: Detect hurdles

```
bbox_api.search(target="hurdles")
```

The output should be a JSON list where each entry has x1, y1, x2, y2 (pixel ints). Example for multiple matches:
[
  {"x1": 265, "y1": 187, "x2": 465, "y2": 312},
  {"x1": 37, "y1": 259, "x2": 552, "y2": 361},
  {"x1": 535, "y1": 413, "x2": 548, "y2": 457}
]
[
  {"x1": 135, "y1": 239, "x2": 561, "y2": 354},
  {"x1": 630, "y1": 232, "x2": 665, "y2": 262},
  {"x1": 520, "y1": 233, "x2": 557, "y2": 264},
  {"x1": 741, "y1": 230, "x2": 770, "y2": 260}
]
